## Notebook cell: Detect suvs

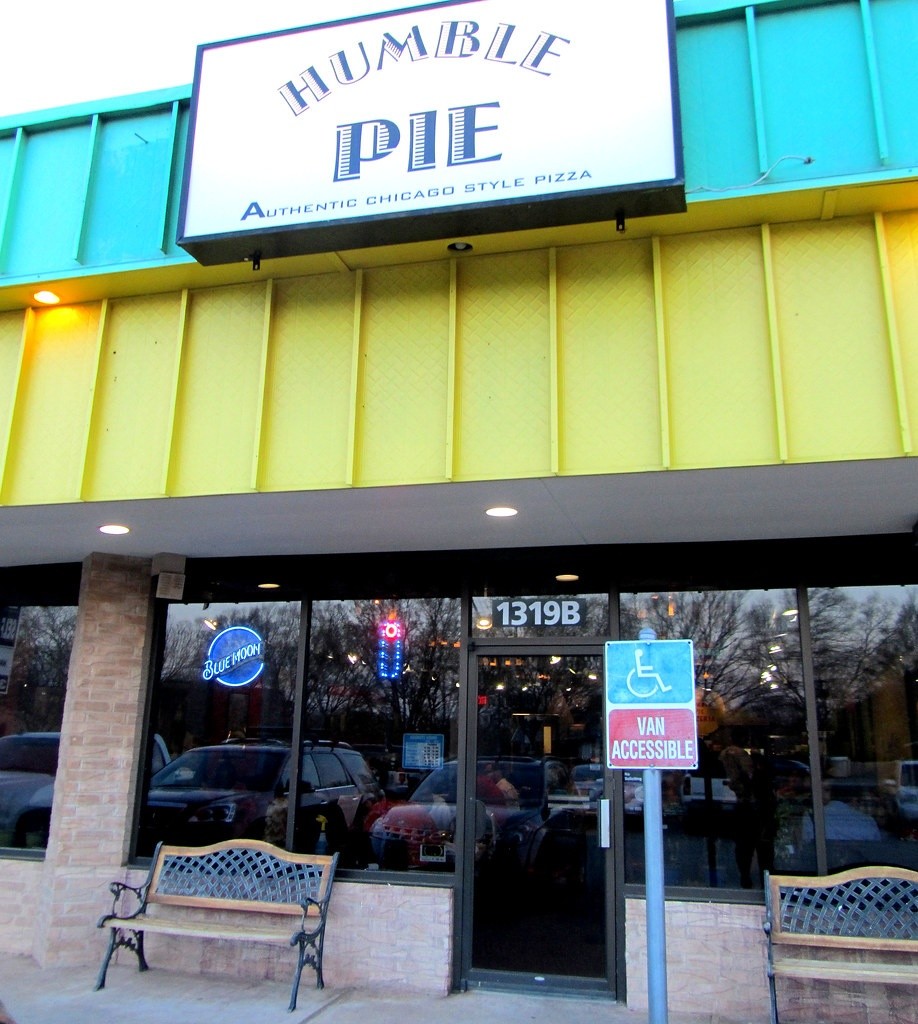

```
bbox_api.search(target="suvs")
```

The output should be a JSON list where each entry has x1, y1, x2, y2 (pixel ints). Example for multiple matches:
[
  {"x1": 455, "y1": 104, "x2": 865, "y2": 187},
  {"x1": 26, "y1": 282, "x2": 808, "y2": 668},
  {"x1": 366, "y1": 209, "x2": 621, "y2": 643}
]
[
  {"x1": 370, "y1": 755, "x2": 587, "y2": 872},
  {"x1": 0, "y1": 732, "x2": 172, "y2": 848},
  {"x1": 144, "y1": 737, "x2": 389, "y2": 868}
]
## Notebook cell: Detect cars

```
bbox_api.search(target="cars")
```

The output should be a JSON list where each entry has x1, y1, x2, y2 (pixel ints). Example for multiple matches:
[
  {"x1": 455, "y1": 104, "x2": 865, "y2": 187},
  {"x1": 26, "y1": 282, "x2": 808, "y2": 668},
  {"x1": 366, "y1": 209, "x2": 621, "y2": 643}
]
[{"x1": 222, "y1": 726, "x2": 813, "y2": 827}]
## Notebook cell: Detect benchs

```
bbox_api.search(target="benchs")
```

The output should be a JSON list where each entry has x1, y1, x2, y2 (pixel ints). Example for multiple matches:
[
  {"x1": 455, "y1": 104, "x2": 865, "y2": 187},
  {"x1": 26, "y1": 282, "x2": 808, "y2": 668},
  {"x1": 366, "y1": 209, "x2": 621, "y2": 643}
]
[
  {"x1": 761, "y1": 865, "x2": 918, "y2": 1024},
  {"x1": 95, "y1": 839, "x2": 340, "y2": 1012}
]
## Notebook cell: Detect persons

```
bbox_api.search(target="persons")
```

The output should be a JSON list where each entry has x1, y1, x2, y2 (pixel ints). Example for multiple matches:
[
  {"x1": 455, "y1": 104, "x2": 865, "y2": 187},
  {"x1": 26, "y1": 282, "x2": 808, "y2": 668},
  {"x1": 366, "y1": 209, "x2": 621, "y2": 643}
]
[
  {"x1": 876, "y1": 779, "x2": 909, "y2": 835},
  {"x1": 476, "y1": 763, "x2": 518, "y2": 807},
  {"x1": 803, "y1": 775, "x2": 881, "y2": 845},
  {"x1": 730, "y1": 735, "x2": 809, "y2": 889}
]
